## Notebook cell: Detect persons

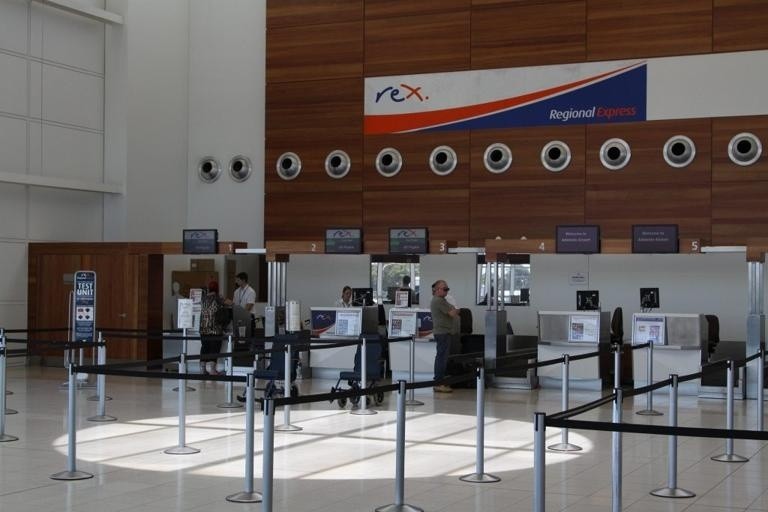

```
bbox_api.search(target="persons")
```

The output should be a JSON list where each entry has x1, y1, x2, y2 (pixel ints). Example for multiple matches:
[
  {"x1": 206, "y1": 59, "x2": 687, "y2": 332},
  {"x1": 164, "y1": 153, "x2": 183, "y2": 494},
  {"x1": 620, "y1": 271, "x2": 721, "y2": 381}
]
[
  {"x1": 431, "y1": 279, "x2": 459, "y2": 393},
  {"x1": 395, "y1": 276, "x2": 416, "y2": 304},
  {"x1": 232, "y1": 271, "x2": 256, "y2": 329},
  {"x1": 200, "y1": 282, "x2": 228, "y2": 376},
  {"x1": 337, "y1": 286, "x2": 352, "y2": 307}
]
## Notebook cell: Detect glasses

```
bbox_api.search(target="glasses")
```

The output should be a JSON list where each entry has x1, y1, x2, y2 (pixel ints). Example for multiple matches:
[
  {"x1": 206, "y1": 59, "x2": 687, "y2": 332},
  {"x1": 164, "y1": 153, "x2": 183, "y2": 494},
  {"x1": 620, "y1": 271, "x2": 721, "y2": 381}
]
[{"x1": 438, "y1": 287, "x2": 450, "y2": 291}]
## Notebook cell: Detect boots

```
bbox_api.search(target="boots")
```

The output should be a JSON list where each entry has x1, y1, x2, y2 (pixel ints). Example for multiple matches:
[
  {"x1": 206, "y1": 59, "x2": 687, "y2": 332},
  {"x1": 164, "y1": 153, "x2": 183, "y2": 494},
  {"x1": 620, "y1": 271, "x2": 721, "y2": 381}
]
[{"x1": 200, "y1": 361, "x2": 218, "y2": 375}]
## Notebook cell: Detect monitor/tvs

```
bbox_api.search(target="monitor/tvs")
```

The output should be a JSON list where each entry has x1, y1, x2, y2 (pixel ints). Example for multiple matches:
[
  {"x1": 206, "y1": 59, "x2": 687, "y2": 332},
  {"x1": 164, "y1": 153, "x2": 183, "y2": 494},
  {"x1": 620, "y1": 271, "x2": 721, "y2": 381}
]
[
  {"x1": 388, "y1": 287, "x2": 399, "y2": 299},
  {"x1": 352, "y1": 288, "x2": 373, "y2": 306},
  {"x1": 577, "y1": 291, "x2": 599, "y2": 310},
  {"x1": 202, "y1": 287, "x2": 208, "y2": 298},
  {"x1": 640, "y1": 288, "x2": 659, "y2": 308},
  {"x1": 521, "y1": 289, "x2": 529, "y2": 301}
]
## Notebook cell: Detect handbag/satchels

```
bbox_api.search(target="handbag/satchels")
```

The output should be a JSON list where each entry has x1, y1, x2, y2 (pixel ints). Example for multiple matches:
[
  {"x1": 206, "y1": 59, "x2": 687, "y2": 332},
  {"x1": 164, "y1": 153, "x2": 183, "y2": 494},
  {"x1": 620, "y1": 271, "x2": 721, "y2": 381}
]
[{"x1": 215, "y1": 308, "x2": 233, "y2": 326}]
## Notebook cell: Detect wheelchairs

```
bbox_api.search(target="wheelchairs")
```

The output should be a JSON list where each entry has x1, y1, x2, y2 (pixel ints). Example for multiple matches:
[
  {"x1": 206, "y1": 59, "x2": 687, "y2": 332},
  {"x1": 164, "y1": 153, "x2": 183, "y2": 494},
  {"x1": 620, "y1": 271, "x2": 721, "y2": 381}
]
[
  {"x1": 237, "y1": 334, "x2": 300, "y2": 403},
  {"x1": 329, "y1": 334, "x2": 386, "y2": 408}
]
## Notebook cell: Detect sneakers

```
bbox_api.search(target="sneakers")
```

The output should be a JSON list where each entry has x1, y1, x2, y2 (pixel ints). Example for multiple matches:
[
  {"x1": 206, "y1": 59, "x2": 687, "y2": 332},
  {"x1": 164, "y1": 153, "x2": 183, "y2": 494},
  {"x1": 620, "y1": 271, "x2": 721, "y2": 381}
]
[{"x1": 433, "y1": 385, "x2": 453, "y2": 393}]
